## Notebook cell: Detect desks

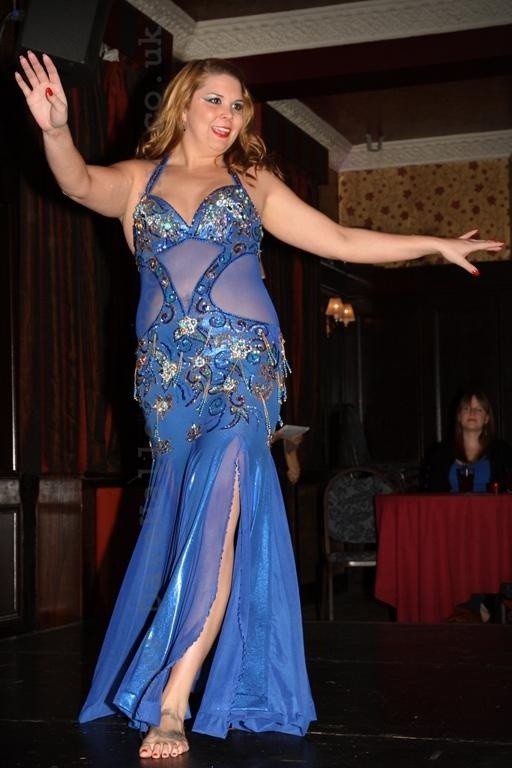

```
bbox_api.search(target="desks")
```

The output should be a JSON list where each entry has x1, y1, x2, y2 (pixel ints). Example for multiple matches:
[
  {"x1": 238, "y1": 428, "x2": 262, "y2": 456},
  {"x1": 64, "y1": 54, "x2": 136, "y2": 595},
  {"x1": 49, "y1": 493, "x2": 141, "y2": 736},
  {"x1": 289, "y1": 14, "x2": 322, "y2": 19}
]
[{"x1": 372, "y1": 494, "x2": 510, "y2": 622}]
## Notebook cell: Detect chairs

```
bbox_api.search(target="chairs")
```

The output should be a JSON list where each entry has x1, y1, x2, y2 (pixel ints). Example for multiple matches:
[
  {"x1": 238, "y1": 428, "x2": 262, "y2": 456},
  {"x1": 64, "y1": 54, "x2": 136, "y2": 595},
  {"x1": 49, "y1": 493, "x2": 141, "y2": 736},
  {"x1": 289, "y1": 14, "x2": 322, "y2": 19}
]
[{"x1": 322, "y1": 468, "x2": 400, "y2": 619}]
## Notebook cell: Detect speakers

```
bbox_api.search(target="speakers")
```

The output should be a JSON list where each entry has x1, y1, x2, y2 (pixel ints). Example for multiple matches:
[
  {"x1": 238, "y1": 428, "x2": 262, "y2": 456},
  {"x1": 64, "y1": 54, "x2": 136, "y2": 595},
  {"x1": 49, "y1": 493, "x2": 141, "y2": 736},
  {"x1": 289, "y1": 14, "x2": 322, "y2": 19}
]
[{"x1": 16, "y1": 0, "x2": 113, "y2": 71}]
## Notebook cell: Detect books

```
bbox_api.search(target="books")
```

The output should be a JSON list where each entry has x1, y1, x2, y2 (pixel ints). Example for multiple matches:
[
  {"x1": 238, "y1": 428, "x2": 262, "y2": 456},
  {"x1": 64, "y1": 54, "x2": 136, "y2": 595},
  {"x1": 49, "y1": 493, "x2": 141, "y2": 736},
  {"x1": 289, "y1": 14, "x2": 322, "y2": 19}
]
[{"x1": 278, "y1": 424, "x2": 311, "y2": 439}]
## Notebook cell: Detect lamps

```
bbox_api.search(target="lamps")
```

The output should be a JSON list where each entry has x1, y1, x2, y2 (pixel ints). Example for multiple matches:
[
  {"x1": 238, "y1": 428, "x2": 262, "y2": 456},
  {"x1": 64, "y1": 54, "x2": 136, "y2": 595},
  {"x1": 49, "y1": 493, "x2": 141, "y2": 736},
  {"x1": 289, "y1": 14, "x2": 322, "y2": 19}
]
[{"x1": 322, "y1": 296, "x2": 356, "y2": 338}]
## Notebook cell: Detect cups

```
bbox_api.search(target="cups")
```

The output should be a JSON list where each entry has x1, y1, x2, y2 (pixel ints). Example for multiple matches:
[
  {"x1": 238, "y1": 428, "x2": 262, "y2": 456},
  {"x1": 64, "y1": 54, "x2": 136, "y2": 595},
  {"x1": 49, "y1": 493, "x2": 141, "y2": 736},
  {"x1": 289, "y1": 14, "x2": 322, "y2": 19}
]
[{"x1": 456, "y1": 465, "x2": 475, "y2": 493}]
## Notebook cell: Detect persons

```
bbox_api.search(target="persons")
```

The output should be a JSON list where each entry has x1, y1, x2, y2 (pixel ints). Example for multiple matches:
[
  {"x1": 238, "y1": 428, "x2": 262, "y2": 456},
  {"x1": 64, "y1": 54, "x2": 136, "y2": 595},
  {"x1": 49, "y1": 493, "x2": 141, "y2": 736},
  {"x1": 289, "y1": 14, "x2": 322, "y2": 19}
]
[
  {"x1": 270, "y1": 430, "x2": 303, "y2": 486},
  {"x1": 13, "y1": 48, "x2": 506, "y2": 759},
  {"x1": 424, "y1": 390, "x2": 500, "y2": 498}
]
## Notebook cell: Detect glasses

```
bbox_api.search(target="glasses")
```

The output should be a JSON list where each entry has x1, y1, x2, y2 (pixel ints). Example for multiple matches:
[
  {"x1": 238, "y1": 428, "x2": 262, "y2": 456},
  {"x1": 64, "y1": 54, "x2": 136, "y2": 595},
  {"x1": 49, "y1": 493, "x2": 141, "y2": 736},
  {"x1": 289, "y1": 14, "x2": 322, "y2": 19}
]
[{"x1": 461, "y1": 407, "x2": 484, "y2": 413}]
